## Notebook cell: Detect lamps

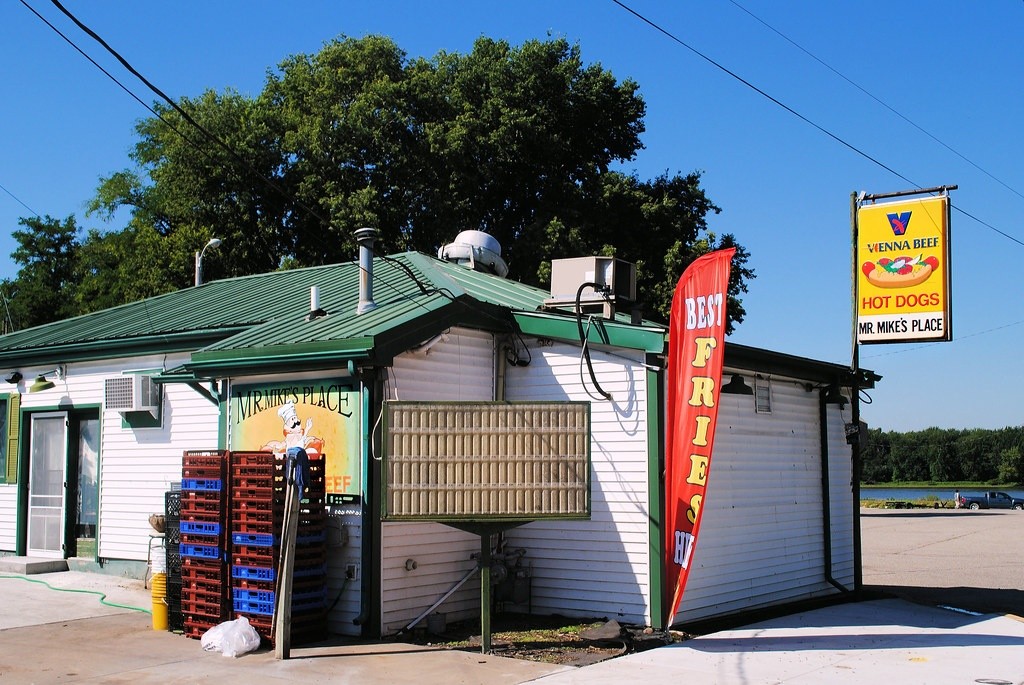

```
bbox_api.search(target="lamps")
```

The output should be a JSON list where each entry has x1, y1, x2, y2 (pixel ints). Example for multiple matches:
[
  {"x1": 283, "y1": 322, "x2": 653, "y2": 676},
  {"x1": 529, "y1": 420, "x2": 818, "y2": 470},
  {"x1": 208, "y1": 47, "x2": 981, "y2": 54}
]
[
  {"x1": 29, "y1": 367, "x2": 63, "y2": 393},
  {"x1": 722, "y1": 374, "x2": 754, "y2": 396},
  {"x1": 824, "y1": 387, "x2": 848, "y2": 404},
  {"x1": 5, "y1": 371, "x2": 23, "y2": 384}
]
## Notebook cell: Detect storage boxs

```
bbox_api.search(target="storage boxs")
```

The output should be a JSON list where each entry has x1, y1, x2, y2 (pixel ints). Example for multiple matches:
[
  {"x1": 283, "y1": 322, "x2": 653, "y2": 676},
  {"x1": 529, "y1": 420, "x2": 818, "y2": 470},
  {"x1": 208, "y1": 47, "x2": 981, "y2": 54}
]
[{"x1": 164, "y1": 447, "x2": 329, "y2": 650}]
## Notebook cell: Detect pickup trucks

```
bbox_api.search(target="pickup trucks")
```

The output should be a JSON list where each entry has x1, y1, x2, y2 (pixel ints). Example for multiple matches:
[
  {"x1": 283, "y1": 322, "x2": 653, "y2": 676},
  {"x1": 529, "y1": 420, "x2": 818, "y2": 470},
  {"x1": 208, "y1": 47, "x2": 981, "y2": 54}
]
[{"x1": 960, "y1": 490, "x2": 1024, "y2": 511}]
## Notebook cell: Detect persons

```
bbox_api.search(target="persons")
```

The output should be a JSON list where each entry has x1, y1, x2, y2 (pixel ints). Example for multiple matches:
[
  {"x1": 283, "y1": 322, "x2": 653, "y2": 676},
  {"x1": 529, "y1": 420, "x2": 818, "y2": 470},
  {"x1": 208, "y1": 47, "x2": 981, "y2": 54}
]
[{"x1": 955, "y1": 490, "x2": 960, "y2": 509}]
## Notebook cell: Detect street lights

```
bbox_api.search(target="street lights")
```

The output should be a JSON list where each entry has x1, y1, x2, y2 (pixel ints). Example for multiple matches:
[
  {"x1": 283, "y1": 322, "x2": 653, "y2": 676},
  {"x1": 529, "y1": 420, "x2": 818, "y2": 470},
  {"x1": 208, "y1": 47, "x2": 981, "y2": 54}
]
[{"x1": 194, "y1": 238, "x2": 223, "y2": 287}]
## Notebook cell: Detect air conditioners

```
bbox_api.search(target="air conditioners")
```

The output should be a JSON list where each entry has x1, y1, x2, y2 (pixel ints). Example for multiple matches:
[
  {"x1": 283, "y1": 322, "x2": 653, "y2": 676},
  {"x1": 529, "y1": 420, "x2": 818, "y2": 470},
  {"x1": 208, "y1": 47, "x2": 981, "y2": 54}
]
[{"x1": 104, "y1": 375, "x2": 161, "y2": 412}]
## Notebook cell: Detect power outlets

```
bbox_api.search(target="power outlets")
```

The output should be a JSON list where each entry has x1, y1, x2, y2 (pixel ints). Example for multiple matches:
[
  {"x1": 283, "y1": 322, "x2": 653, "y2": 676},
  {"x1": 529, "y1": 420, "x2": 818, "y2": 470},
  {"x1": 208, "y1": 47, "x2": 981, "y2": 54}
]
[{"x1": 345, "y1": 564, "x2": 357, "y2": 582}]
